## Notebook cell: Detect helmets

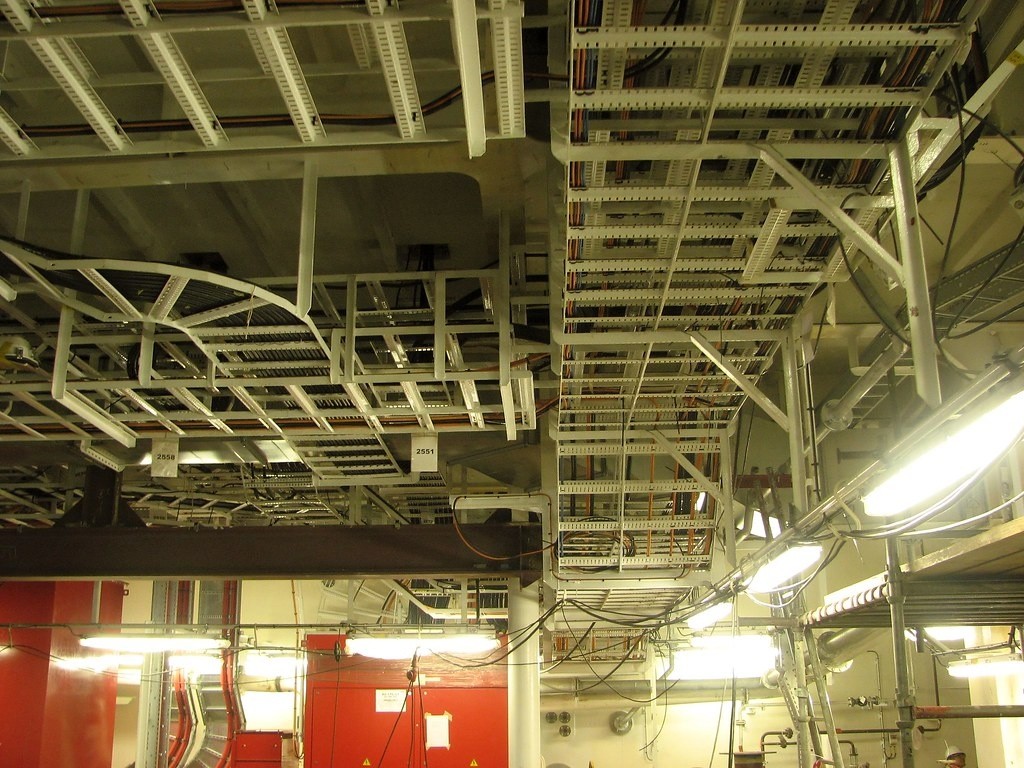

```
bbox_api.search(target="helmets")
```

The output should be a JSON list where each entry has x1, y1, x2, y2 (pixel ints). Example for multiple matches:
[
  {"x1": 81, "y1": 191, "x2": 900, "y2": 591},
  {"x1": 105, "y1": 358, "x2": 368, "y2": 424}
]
[{"x1": 945, "y1": 746, "x2": 966, "y2": 760}]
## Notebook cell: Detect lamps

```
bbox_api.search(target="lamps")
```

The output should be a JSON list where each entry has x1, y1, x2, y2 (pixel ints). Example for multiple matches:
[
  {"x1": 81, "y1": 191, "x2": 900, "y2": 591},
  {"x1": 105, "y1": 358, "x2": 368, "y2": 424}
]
[
  {"x1": 948, "y1": 653, "x2": 1024, "y2": 678},
  {"x1": 80, "y1": 633, "x2": 232, "y2": 653},
  {"x1": 346, "y1": 631, "x2": 500, "y2": 661},
  {"x1": 740, "y1": 541, "x2": 823, "y2": 593}
]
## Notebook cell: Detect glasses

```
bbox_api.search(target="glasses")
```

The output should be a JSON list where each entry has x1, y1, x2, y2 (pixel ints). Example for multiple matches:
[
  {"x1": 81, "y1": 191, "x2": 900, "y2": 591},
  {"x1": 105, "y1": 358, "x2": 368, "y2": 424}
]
[{"x1": 950, "y1": 753, "x2": 965, "y2": 760}]
